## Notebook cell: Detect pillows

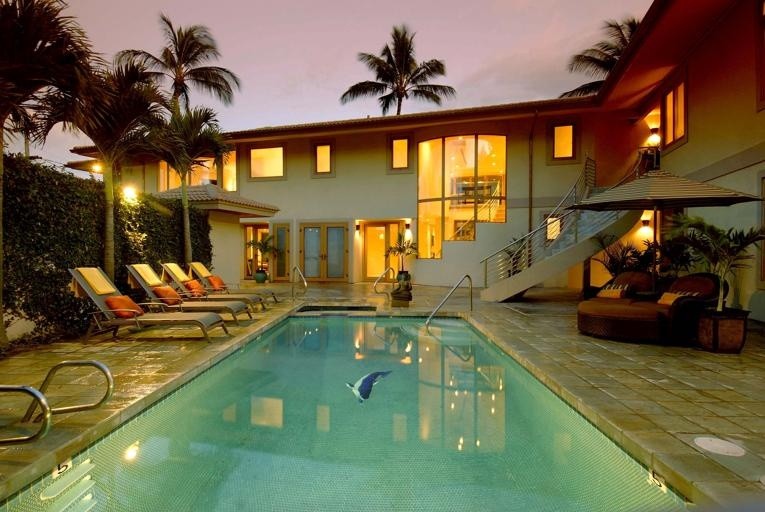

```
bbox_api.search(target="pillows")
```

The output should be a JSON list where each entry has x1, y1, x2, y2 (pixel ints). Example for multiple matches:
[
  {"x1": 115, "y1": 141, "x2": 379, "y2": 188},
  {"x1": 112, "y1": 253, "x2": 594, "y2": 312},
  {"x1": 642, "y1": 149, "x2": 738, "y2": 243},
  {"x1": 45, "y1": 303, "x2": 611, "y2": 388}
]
[
  {"x1": 597, "y1": 284, "x2": 630, "y2": 300},
  {"x1": 657, "y1": 288, "x2": 702, "y2": 306}
]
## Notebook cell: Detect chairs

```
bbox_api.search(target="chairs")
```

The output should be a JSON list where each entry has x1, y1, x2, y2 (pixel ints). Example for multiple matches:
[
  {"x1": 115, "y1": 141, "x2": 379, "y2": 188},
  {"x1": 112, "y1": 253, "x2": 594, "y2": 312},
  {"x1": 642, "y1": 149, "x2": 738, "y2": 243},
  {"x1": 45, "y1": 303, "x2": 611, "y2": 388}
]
[{"x1": 64, "y1": 257, "x2": 282, "y2": 347}]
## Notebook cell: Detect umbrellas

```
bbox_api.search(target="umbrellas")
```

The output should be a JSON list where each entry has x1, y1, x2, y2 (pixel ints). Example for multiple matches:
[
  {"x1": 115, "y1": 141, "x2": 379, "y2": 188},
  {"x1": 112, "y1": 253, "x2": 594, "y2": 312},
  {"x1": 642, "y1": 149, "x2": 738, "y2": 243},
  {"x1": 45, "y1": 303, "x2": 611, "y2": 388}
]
[{"x1": 562, "y1": 164, "x2": 765, "y2": 303}]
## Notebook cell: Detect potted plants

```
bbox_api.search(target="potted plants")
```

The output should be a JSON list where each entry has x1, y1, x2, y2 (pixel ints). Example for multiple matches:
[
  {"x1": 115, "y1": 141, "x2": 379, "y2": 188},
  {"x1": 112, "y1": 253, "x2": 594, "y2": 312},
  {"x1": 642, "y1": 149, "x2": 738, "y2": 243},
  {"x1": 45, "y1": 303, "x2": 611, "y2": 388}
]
[
  {"x1": 660, "y1": 209, "x2": 764, "y2": 355},
  {"x1": 245, "y1": 234, "x2": 282, "y2": 285},
  {"x1": 383, "y1": 229, "x2": 420, "y2": 281}
]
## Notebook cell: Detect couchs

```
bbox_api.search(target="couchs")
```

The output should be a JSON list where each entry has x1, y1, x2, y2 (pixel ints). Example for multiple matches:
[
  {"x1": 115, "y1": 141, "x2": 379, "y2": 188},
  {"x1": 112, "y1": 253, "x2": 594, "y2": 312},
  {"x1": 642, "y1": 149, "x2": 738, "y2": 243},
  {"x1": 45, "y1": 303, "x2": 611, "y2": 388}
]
[{"x1": 575, "y1": 267, "x2": 728, "y2": 349}]
